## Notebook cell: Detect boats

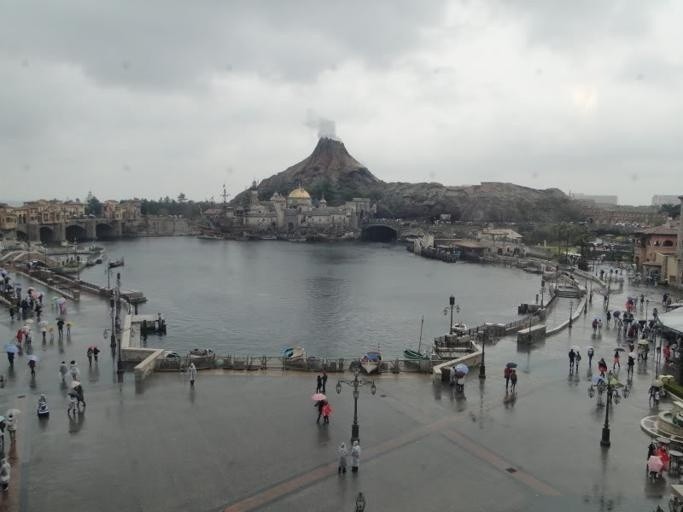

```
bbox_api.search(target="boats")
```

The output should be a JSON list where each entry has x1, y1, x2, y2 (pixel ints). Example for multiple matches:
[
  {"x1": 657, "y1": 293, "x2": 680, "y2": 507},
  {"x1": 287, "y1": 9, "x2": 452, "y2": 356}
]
[
  {"x1": 194, "y1": 184, "x2": 362, "y2": 242},
  {"x1": 450, "y1": 323, "x2": 467, "y2": 333},
  {"x1": 277, "y1": 343, "x2": 304, "y2": 367},
  {"x1": 359, "y1": 350, "x2": 383, "y2": 375},
  {"x1": 164, "y1": 351, "x2": 181, "y2": 369},
  {"x1": 187, "y1": 347, "x2": 214, "y2": 366},
  {"x1": 401, "y1": 347, "x2": 431, "y2": 368},
  {"x1": 107, "y1": 256, "x2": 124, "y2": 268}
]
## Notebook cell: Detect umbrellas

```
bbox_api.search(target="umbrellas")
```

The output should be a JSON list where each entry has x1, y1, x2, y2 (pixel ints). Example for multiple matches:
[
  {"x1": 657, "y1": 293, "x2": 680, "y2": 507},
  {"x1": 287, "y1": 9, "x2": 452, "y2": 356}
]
[
  {"x1": 654, "y1": 447, "x2": 669, "y2": 464},
  {"x1": 311, "y1": 392, "x2": 326, "y2": 401},
  {"x1": 456, "y1": 363, "x2": 468, "y2": 374},
  {"x1": 647, "y1": 455, "x2": 663, "y2": 473},
  {"x1": 505, "y1": 361, "x2": 517, "y2": 368}
]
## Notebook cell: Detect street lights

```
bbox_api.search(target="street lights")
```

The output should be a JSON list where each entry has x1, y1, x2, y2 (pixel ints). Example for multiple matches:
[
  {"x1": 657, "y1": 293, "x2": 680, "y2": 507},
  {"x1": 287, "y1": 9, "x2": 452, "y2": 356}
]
[
  {"x1": 101, "y1": 273, "x2": 134, "y2": 379},
  {"x1": 529, "y1": 231, "x2": 650, "y2": 332},
  {"x1": 334, "y1": 372, "x2": 376, "y2": 449},
  {"x1": 442, "y1": 294, "x2": 463, "y2": 333},
  {"x1": 583, "y1": 368, "x2": 629, "y2": 450},
  {"x1": 472, "y1": 324, "x2": 498, "y2": 380},
  {"x1": 23, "y1": 233, "x2": 80, "y2": 282}
]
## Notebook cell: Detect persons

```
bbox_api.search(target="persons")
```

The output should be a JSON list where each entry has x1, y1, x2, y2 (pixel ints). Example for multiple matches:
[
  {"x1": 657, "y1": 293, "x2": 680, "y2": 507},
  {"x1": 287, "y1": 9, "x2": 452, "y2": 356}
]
[
  {"x1": 503, "y1": 367, "x2": 512, "y2": 390},
  {"x1": 0, "y1": 263, "x2": 124, "y2": 490},
  {"x1": 647, "y1": 440, "x2": 656, "y2": 459},
  {"x1": 355, "y1": 490, "x2": 366, "y2": 512},
  {"x1": 347, "y1": 440, "x2": 361, "y2": 472},
  {"x1": 314, "y1": 374, "x2": 322, "y2": 392},
  {"x1": 312, "y1": 399, "x2": 327, "y2": 423},
  {"x1": 509, "y1": 368, "x2": 517, "y2": 392},
  {"x1": 448, "y1": 366, "x2": 455, "y2": 384},
  {"x1": 321, "y1": 401, "x2": 332, "y2": 424},
  {"x1": 335, "y1": 440, "x2": 349, "y2": 473},
  {"x1": 319, "y1": 370, "x2": 328, "y2": 393},
  {"x1": 554, "y1": 260, "x2": 682, "y2": 409},
  {"x1": 457, "y1": 375, "x2": 464, "y2": 391},
  {"x1": 186, "y1": 361, "x2": 196, "y2": 386}
]
